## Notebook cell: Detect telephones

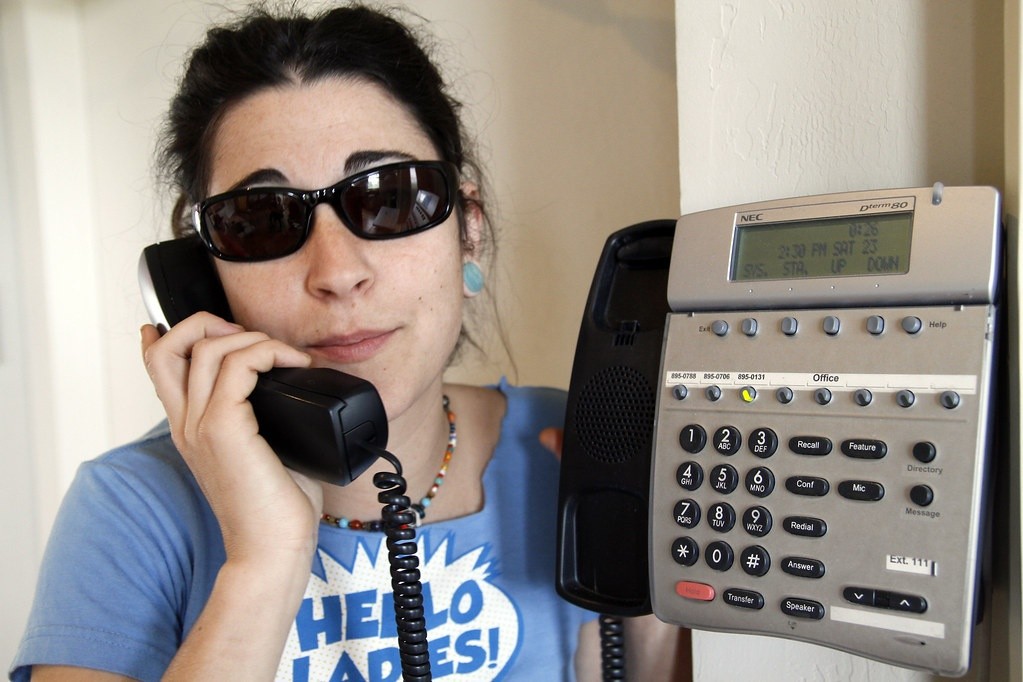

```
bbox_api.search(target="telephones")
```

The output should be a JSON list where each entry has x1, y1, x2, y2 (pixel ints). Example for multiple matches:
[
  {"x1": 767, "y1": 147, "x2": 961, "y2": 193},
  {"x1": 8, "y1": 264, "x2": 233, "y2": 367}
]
[{"x1": 136, "y1": 233, "x2": 389, "y2": 489}]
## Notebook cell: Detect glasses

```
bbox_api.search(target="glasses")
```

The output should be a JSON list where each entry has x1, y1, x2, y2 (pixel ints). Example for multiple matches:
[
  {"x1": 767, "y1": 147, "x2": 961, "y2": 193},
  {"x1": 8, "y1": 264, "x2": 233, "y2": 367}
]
[{"x1": 183, "y1": 159, "x2": 460, "y2": 264}]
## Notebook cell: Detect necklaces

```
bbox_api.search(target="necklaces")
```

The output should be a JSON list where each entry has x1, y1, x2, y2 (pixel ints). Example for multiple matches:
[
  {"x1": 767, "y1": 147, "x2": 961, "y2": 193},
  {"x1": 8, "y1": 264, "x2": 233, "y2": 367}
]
[{"x1": 317, "y1": 393, "x2": 458, "y2": 532}]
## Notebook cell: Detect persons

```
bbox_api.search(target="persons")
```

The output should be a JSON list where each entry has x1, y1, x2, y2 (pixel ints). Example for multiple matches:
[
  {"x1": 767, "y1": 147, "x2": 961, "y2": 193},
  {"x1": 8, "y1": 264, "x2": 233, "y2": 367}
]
[{"x1": 7, "y1": 0, "x2": 678, "y2": 682}]
